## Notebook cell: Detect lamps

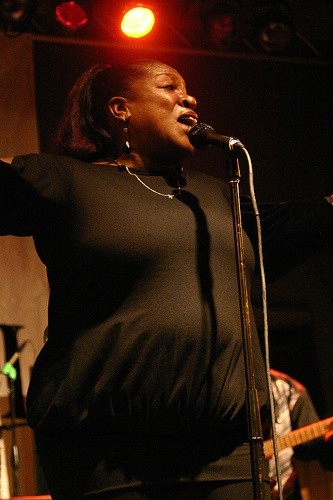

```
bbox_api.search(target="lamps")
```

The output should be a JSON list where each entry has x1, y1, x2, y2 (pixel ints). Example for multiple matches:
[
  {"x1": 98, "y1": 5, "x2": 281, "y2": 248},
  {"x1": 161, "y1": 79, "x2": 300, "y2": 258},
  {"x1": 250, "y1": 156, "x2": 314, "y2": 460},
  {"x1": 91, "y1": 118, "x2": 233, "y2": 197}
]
[
  {"x1": 200, "y1": 2, "x2": 240, "y2": 49},
  {"x1": 248, "y1": 0, "x2": 293, "y2": 60},
  {"x1": 0, "y1": 0, "x2": 94, "y2": 39},
  {"x1": 116, "y1": 1, "x2": 156, "y2": 40}
]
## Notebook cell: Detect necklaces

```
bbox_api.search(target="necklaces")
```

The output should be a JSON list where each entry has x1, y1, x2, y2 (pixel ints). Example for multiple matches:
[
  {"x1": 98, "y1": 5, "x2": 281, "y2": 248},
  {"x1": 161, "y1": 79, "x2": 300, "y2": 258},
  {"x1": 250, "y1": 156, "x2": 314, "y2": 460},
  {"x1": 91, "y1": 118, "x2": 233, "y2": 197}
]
[{"x1": 114, "y1": 159, "x2": 182, "y2": 199}]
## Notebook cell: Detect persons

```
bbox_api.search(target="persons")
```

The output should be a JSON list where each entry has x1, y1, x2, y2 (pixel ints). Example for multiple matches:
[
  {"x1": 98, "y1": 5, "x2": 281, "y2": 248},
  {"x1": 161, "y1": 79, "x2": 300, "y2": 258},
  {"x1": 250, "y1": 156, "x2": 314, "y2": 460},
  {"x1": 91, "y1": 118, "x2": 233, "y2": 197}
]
[{"x1": 0, "y1": 58, "x2": 333, "y2": 500}]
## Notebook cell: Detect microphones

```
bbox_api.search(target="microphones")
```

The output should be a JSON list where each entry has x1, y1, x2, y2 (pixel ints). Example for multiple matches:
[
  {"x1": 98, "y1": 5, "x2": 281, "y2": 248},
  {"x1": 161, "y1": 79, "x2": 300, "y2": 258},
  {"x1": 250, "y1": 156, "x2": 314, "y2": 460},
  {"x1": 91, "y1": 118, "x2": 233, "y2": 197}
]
[{"x1": 188, "y1": 122, "x2": 246, "y2": 152}]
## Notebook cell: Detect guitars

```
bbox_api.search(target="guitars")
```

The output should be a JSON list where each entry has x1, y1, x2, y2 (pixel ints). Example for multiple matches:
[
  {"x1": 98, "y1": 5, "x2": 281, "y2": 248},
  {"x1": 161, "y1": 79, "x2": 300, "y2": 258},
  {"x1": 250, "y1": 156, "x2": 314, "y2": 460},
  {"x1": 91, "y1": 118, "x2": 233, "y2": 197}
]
[{"x1": 263, "y1": 416, "x2": 333, "y2": 460}]
